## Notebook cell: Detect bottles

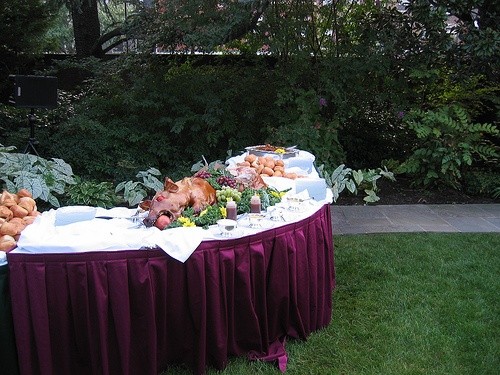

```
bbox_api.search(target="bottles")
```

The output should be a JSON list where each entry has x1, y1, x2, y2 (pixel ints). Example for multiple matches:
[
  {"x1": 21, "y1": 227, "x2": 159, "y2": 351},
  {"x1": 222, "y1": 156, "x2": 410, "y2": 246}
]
[
  {"x1": 250, "y1": 192, "x2": 261, "y2": 213},
  {"x1": 226, "y1": 201, "x2": 237, "y2": 220}
]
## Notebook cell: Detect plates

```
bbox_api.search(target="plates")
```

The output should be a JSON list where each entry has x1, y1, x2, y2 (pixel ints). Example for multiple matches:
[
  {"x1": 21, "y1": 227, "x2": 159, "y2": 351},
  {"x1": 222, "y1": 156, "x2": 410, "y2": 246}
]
[{"x1": 263, "y1": 176, "x2": 295, "y2": 191}]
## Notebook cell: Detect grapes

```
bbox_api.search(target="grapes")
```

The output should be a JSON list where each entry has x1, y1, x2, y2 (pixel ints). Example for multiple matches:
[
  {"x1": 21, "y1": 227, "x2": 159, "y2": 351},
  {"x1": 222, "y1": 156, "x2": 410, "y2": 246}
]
[
  {"x1": 216, "y1": 175, "x2": 237, "y2": 188},
  {"x1": 193, "y1": 170, "x2": 212, "y2": 179},
  {"x1": 215, "y1": 185, "x2": 242, "y2": 202}
]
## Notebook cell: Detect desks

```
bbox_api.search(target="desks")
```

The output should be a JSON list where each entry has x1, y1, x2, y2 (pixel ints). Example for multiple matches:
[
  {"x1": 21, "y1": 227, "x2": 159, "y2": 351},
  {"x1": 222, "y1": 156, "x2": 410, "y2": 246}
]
[{"x1": 7, "y1": 148, "x2": 336, "y2": 375}]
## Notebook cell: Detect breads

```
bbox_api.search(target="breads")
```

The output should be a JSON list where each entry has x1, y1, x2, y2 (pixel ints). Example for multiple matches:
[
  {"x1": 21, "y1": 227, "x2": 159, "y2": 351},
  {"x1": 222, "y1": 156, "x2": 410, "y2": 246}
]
[
  {"x1": 0, "y1": 188, "x2": 42, "y2": 252},
  {"x1": 237, "y1": 154, "x2": 297, "y2": 179}
]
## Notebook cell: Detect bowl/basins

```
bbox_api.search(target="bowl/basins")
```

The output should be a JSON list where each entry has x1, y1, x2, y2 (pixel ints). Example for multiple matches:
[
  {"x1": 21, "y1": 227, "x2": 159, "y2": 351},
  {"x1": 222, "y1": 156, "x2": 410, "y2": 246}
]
[
  {"x1": 248, "y1": 214, "x2": 266, "y2": 229},
  {"x1": 216, "y1": 219, "x2": 237, "y2": 236},
  {"x1": 267, "y1": 205, "x2": 285, "y2": 220},
  {"x1": 286, "y1": 197, "x2": 304, "y2": 211}
]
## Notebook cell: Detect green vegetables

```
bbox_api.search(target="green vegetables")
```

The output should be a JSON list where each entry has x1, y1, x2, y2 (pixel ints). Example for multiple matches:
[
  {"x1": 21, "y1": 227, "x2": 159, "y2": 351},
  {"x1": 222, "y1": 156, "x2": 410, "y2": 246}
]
[{"x1": 164, "y1": 165, "x2": 285, "y2": 231}]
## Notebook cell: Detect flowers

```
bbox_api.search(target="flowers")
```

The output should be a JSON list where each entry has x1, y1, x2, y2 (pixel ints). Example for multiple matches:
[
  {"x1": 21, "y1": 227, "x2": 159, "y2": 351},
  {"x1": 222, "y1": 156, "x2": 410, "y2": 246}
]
[{"x1": 165, "y1": 185, "x2": 292, "y2": 230}]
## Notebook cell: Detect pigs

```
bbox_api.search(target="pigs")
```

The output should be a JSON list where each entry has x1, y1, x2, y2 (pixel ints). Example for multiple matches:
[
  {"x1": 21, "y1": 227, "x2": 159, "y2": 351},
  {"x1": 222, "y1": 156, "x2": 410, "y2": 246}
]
[{"x1": 138, "y1": 176, "x2": 216, "y2": 228}]
